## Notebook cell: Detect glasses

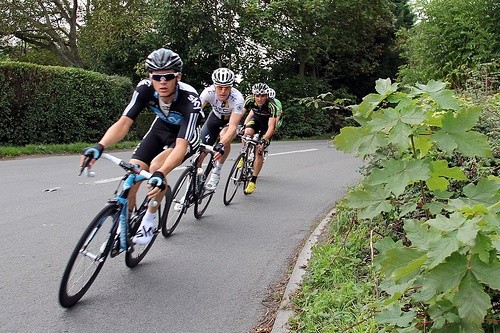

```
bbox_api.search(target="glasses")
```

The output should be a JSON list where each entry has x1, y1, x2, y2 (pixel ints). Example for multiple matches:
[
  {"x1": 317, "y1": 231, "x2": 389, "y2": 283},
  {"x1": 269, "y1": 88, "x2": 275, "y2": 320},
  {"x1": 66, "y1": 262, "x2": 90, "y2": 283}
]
[
  {"x1": 148, "y1": 73, "x2": 179, "y2": 81},
  {"x1": 216, "y1": 86, "x2": 231, "y2": 90}
]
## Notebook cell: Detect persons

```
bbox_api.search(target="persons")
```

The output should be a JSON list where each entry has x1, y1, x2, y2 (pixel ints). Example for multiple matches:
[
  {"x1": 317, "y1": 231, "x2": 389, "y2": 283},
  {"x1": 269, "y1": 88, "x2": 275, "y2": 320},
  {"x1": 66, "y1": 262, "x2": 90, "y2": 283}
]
[
  {"x1": 80, "y1": 48, "x2": 202, "y2": 254},
  {"x1": 173, "y1": 68, "x2": 245, "y2": 213},
  {"x1": 236, "y1": 83, "x2": 284, "y2": 194}
]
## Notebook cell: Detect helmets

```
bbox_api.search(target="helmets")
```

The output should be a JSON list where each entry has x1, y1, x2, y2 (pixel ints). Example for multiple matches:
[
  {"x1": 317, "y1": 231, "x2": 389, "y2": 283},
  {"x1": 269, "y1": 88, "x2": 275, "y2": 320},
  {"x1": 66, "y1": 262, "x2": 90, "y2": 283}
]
[
  {"x1": 252, "y1": 83, "x2": 269, "y2": 98},
  {"x1": 211, "y1": 68, "x2": 234, "y2": 87},
  {"x1": 269, "y1": 88, "x2": 276, "y2": 99},
  {"x1": 144, "y1": 48, "x2": 183, "y2": 72}
]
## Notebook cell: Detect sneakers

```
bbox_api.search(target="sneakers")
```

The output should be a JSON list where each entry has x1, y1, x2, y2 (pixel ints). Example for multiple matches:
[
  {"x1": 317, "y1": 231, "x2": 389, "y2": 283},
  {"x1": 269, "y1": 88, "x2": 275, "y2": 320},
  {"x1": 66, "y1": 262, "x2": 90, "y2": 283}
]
[
  {"x1": 174, "y1": 197, "x2": 185, "y2": 212},
  {"x1": 244, "y1": 182, "x2": 256, "y2": 194},
  {"x1": 206, "y1": 174, "x2": 220, "y2": 191},
  {"x1": 237, "y1": 157, "x2": 244, "y2": 168},
  {"x1": 132, "y1": 216, "x2": 159, "y2": 244}
]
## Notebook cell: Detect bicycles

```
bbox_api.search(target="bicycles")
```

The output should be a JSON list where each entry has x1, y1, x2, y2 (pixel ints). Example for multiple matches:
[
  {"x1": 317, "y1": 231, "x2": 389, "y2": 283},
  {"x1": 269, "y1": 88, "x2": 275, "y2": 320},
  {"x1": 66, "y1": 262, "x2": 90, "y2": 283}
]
[
  {"x1": 161, "y1": 130, "x2": 225, "y2": 238},
  {"x1": 223, "y1": 132, "x2": 271, "y2": 205},
  {"x1": 57, "y1": 147, "x2": 173, "y2": 309}
]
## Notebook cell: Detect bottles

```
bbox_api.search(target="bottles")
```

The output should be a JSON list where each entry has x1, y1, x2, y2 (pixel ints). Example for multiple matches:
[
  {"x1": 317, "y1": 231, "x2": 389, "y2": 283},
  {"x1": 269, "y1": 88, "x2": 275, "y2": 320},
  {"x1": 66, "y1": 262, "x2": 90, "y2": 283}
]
[{"x1": 198, "y1": 168, "x2": 203, "y2": 185}]
